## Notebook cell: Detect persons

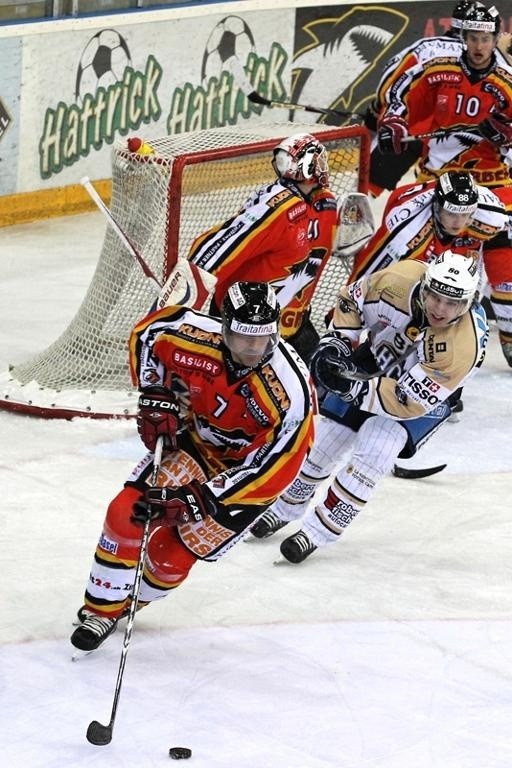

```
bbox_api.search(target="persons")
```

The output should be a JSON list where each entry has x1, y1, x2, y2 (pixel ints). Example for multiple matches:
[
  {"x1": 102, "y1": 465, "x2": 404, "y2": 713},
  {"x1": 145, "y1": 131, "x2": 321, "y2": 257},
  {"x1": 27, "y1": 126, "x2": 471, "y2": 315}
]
[
  {"x1": 136, "y1": 0, "x2": 512, "y2": 567},
  {"x1": 63, "y1": 279, "x2": 323, "y2": 655}
]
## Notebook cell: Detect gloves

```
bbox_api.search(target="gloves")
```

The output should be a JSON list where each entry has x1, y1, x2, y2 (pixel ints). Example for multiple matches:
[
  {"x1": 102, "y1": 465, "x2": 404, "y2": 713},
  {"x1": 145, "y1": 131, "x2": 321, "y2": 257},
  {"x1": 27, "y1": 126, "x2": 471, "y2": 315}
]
[
  {"x1": 308, "y1": 331, "x2": 369, "y2": 408},
  {"x1": 378, "y1": 113, "x2": 411, "y2": 156},
  {"x1": 129, "y1": 479, "x2": 218, "y2": 530},
  {"x1": 477, "y1": 111, "x2": 511, "y2": 149},
  {"x1": 138, "y1": 384, "x2": 179, "y2": 453}
]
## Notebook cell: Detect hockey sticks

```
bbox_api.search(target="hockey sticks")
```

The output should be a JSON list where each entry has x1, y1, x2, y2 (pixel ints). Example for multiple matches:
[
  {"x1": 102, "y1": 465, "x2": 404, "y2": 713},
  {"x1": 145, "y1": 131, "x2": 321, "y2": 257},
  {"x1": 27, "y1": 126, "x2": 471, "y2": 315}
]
[
  {"x1": 85, "y1": 435, "x2": 163, "y2": 746},
  {"x1": 390, "y1": 462, "x2": 448, "y2": 480},
  {"x1": 230, "y1": 57, "x2": 366, "y2": 120}
]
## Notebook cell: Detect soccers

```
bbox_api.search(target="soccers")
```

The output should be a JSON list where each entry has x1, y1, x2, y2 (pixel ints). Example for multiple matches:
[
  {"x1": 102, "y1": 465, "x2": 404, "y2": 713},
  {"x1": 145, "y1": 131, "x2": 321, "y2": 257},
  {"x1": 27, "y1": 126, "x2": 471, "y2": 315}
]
[
  {"x1": 74, "y1": 29, "x2": 133, "y2": 109},
  {"x1": 200, "y1": 15, "x2": 256, "y2": 84}
]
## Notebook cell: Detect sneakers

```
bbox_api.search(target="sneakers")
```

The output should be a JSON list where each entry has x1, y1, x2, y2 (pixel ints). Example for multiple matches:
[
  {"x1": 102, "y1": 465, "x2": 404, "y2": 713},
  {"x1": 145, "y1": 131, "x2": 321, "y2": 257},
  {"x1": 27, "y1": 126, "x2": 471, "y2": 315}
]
[
  {"x1": 71, "y1": 602, "x2": 128, "y2": 650},
  {"x1": 249, "y1": 508, "x2": 289, "y2": 538},
  {"x1": 280, "y1": 527, "x2": 319, "y2": 565}
]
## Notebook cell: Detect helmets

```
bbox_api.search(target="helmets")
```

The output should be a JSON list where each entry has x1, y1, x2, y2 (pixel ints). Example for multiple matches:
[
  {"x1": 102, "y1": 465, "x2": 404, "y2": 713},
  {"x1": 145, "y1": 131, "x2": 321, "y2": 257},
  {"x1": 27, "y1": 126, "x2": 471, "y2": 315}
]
[
  {"x1": 269, "y1": 134, "x2": 330, "y2": 192},
  {"x1": 451, "y1": 1, "x2": 501, "y2": 53},
  {"x1": 434, "y1": 169, "x2": 479, "y2": 229},
  {"x1": 220, "y1": 280, "x2": 282, "y2": 357},
  {"x1": 418, "y1": 249, "x2": 480, "y2": 328}
]
